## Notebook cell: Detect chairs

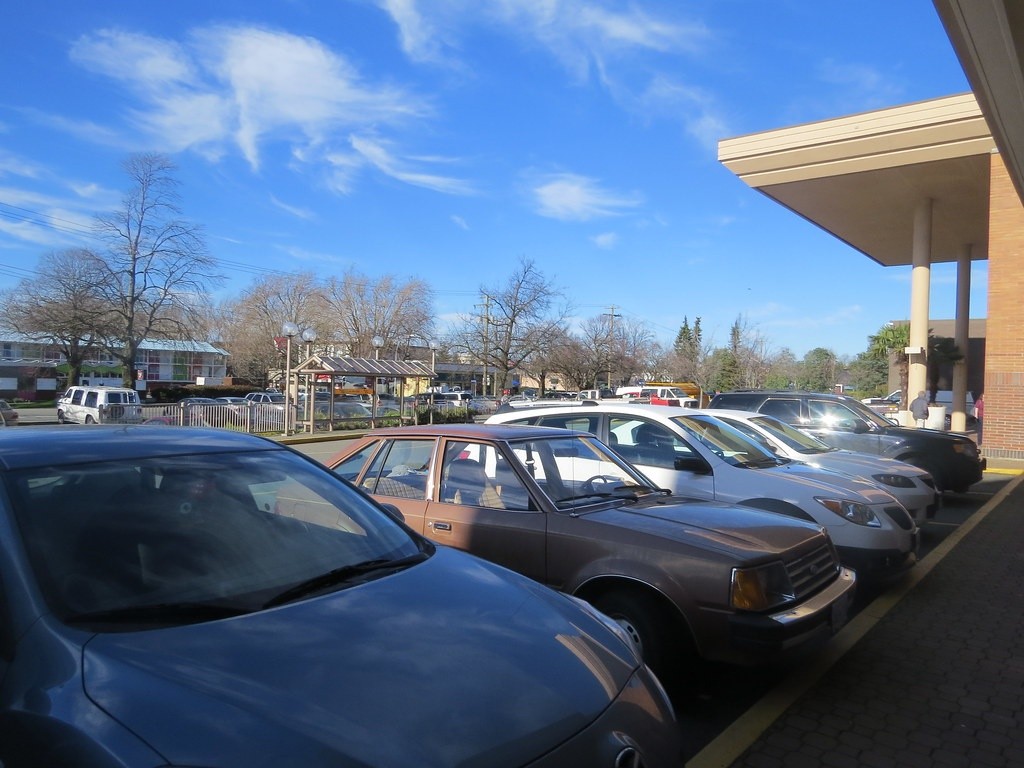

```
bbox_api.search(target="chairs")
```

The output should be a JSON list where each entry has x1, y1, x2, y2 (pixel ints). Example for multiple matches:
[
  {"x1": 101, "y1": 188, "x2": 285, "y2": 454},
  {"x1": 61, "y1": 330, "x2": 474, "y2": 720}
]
[
  {"x1": 498, "y1": 461, "x2": 530, "y2": 511},
  {"x1": 632, "y1": 422, "x2": 672, "y2": 460},
  {"x1": 444, "y1": 459, "x2": 507, "y2": 507}
]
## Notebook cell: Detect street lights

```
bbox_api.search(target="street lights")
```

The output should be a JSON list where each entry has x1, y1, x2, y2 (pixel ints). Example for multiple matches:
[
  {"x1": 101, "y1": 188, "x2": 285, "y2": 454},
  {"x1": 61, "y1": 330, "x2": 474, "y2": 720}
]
[
  {"x1": 428, "y1": 339, "x2": 440, "y2": 425},
  {"x1": 302, "y1": 328, "x2": 316, "y2": 432},
  {"x1": 371, "y1": 335, "x2": 386, "y2": 416},
  {"x1": 281, "y1": 320, "x2": 297, "y2": 437}
]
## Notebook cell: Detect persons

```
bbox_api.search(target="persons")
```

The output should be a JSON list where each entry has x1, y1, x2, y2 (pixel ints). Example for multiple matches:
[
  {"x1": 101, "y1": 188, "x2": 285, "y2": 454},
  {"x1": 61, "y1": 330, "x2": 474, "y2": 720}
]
[
  {"x1": 500, "y1": 389, "x2": 510, "y2": 408},
  {"x1": 909, "y1": 391, "x2": 929, "y2": 429},
  {"x1": 974, "y1": 393, "x2": 984, "y2": 444}
]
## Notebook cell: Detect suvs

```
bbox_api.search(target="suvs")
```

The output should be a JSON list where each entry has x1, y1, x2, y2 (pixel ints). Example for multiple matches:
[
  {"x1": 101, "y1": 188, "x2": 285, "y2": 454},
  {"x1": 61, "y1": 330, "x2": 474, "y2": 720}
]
[
  {"x1": 865, "y1": 388, "x2": 980, "y2": 430},
  {"x1": 246, "y1": 392, "x2": 306, "y2": 418},
  {"x1": 640, "y1": 387, "x2": 699, "y2": 409},
  {"x1": 441, "y1": 391, "x2": 474, "y2": 408},
  {"x1": 57, "y1": 386, "x2": 143, "y2": 424},
  {"x1": 706, "y1": 390, "x2": 988, "y2": 496}
]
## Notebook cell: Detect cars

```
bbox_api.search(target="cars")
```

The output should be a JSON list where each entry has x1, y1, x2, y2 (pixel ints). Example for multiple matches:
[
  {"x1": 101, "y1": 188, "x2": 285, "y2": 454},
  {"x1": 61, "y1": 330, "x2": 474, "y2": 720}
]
[
  {"x1": 275, "y1": 423, "x2": 856, "y2": 690},
  {"x1": 266, "y1": 387, "x2": 449, "y2": 422},
  {"x1": 449, "y1": 399, "x2": 922, "y2": 584},
  {"x1": 143, "y1": 417, "x2": 198, "y2": 426},
  {"x1": 0, "y1": 400, "x2": 19, "y2": 425},
  {"x1": 503, "y1": 385, "x2": 640, "y2": 406},
  {"x1": 162, "y1": 397, "x2": 236, "y2": 428},
  {"x1": 0, "y1": 424, "x2": 678, "y2": 766},
  {"x1": 216, "y1": 396, "x2": 257, "y2": 421},
  {"x1": 611, "y1": 408, "x2": 944, "y2": 525}
]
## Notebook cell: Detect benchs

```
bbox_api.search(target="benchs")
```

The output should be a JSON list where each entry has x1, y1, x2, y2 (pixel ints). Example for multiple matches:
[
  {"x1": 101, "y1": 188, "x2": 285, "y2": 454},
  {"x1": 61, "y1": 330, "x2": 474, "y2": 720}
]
[{"x1": 364, "y1": 474, "x2": 436, "y2": 503}]
